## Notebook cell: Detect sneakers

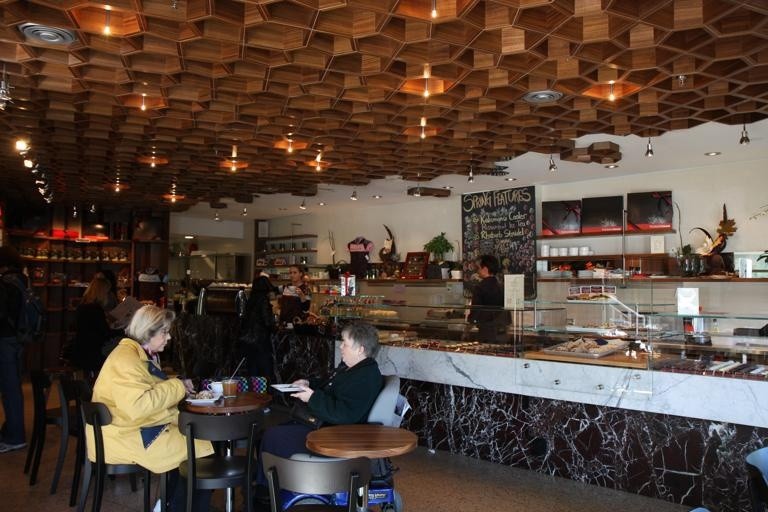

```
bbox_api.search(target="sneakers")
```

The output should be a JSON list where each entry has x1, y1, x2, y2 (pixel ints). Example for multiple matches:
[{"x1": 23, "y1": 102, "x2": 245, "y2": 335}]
[{"x1": 0, "y1": 442, "x2": 28, "y2": 453}]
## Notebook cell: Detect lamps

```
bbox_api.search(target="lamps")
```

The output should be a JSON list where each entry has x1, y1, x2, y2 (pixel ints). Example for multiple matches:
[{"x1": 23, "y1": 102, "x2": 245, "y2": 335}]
[{"x1": 0, "y1": 1, "x2": 768, "y2": 224}]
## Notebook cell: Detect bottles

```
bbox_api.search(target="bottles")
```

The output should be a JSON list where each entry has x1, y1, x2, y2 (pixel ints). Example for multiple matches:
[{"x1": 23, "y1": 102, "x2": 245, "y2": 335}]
[
  {"x1": 22, "y1": 247, "x2": 48, "y2": 259},
  {"x1": 712, "y1": 319, "x2": 720, "y2": 335},
  {"x1": 365, "y1": 265, "x2": 376, "y2": 279}
]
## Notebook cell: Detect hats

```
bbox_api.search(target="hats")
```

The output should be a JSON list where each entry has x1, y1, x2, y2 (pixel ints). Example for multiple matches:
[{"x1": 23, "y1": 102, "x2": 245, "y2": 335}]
[{"x1": 0, "y1": 245, "x2": 19, "y2": 268}]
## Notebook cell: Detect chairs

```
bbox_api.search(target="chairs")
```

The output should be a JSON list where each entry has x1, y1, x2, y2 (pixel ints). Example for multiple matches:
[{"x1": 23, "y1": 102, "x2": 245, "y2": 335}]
[{"x1": 24, "y1": 285, "x2": 418, "y2": 512}]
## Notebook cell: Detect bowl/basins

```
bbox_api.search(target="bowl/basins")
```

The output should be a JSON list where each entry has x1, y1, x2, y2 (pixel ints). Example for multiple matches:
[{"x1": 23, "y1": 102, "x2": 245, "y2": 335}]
[{"x1": 578, "y1": 270, "x2": 592, "y2": 278}]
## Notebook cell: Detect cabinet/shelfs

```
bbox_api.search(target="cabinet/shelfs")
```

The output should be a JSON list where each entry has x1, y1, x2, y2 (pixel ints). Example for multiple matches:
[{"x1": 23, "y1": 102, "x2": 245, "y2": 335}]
[
  {"x1": 306, "y1": 280, "x2": 767, "y2": 509},
  {"x1": 10, "y1": 229, "x2": 320, "y2": 312}
]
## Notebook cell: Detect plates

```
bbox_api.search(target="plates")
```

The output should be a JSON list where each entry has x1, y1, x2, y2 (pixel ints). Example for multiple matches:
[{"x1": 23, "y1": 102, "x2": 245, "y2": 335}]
[
  {"x1": 185, "y1": 393, "x2": 222, "y2": 404},
  {"x1": 270, "y1": 383, "x2": 302, "y2": 392}
]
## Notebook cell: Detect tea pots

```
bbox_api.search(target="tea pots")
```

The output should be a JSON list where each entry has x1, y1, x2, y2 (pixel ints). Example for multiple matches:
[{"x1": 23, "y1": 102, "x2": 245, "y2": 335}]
[{"x1": 207, "y1": 381, "x2": 223, "y2": 393}]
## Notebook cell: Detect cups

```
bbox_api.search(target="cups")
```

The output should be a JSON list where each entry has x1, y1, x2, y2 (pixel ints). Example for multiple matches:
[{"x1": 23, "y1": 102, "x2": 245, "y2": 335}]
[
  {"x1": 288, "y1": 286, "x2": 297, "y2": 294},
  {"x1": 210, "y1": 281, "x2": 247, "y2": 287},
  {"x1": 450, "y1": 270, "x2": 463, "y2": 279},
  {"x1": 222, "y1": 379, "x2": 239, "y2": 398},
  {"x1": 300, "y1": 256, "x2": 307, "y2": 264},
  {"x1": 288, "y1": 256, "x2": 296, "y2": 265},
  {"x1": 540, "y1": 243, "x2": 589, "y2": 257},
  {"x1": 289, "y1": 242, "x2": 296, "y2": 251},
  {"x1": 302, "y1": 241, "x2": 308, "y2": 251},
  {"x1": 562, "y1": 270, "x2": 572, "y2": 277},
  {"x1": 280, "y1": 243, "x2": 285, "y2": 252}
]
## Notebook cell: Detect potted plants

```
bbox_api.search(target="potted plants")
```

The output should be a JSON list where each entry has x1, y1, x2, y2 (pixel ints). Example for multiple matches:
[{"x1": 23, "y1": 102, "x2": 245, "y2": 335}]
[{"x1": 423, "y1": 232, "x2": 454, "y2": 281}]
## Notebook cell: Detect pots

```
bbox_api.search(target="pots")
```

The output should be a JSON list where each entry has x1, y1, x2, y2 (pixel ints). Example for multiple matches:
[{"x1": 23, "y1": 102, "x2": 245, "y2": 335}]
[
  {"x1": 683, "y1": 330, "x2": 712, "y2": 346},
  {"x1": 679, "y1": 253, "x2": 707, "y2": 275}
]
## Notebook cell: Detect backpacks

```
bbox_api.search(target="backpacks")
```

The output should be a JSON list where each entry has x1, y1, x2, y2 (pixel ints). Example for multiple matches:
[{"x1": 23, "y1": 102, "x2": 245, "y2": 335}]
[{"x1": 3, "y1": 274, "x2": 49, "y2": 345}]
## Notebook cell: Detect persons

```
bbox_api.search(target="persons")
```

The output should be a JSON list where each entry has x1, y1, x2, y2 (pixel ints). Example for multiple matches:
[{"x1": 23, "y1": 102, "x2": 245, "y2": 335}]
[
  {"x1": 462, "y1": 252, "x2": 511, "y2": 347},
  {"x1": 83, "y1": 302, "x2": 216, "y2": 512},
  {"x1": 57, "y1": 271, "x2": 115, "y2": 393},
  {"x1": 233, "y1": 273, "x2": 282, "y2": 376},
  {"x1": 0, "y1": 242, "x2": 31, "y2": 455},
  {"x1": 276, "y1": 262, "x2": 312, "y2": 323},
  {"x1": 247, "y1": 319, "x2": 384, "y2": 511}
]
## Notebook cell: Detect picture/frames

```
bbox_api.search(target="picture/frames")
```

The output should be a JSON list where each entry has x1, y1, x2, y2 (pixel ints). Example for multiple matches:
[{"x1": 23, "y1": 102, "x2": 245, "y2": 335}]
[{"x1": 402, "y1": 252, "x2": 430, "y2": 280}]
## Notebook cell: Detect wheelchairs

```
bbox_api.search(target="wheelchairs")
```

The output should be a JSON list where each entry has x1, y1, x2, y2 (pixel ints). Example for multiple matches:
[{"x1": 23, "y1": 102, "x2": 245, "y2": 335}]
[{"x1": 256, "y1": 365, "x2": 411, "y2": 511}]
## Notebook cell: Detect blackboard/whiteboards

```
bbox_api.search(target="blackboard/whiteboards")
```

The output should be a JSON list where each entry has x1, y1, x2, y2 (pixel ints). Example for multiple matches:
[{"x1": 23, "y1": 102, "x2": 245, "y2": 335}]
[{"x1": 461, "y1": 186, "x2": 537, "y2": 301}]
